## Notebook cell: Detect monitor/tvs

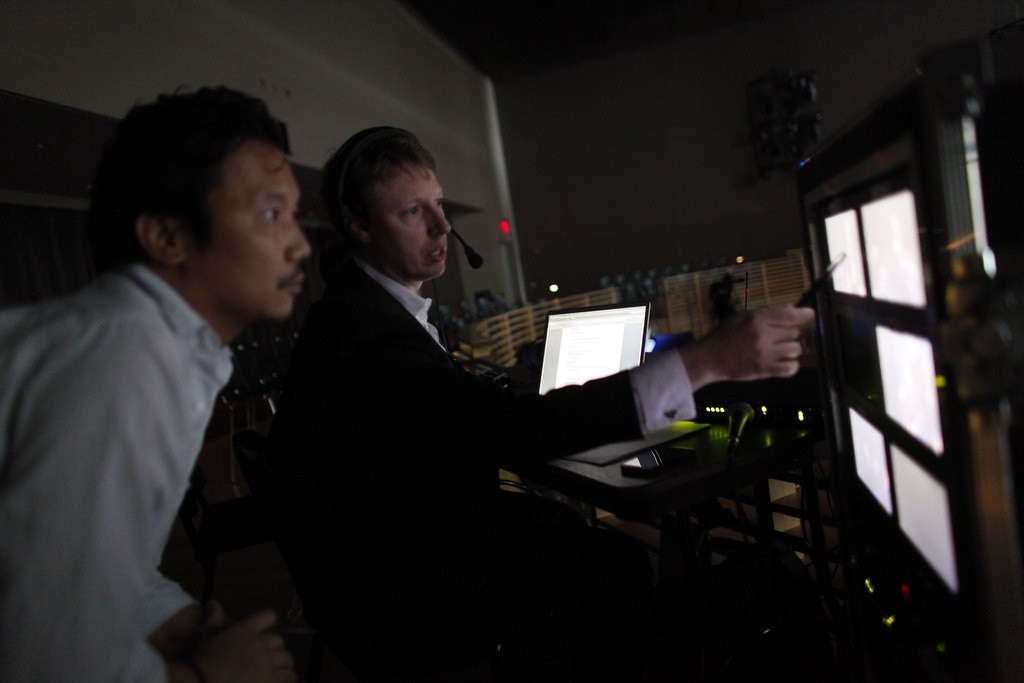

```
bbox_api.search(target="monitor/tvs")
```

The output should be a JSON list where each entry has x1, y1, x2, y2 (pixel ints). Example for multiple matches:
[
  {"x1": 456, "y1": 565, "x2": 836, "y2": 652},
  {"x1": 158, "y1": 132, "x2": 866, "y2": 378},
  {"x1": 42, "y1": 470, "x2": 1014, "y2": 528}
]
[{"x1": 537, "y1": 299, "x2": 652, "y2": 397}]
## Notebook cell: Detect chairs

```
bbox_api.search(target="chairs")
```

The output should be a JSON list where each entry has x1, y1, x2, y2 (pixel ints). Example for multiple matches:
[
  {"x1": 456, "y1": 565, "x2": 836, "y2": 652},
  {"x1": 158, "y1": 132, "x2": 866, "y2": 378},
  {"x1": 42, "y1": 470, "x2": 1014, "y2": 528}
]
[{"x1": 178, "y1": 462, "x2": 327, "y2": 683}]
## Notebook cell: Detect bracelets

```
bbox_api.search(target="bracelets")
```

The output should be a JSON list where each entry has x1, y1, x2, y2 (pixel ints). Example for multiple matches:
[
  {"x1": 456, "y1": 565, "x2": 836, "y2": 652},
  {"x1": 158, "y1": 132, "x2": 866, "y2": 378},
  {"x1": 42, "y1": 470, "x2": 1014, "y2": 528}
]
[{"x1": 180, "y1": 657, "x2": 209, "y2": 682}]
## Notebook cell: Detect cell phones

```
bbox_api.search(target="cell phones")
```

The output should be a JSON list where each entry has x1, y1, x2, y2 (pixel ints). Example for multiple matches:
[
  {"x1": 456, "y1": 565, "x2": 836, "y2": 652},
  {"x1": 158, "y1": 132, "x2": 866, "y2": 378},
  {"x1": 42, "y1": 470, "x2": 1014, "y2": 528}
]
[{"x1": 621, "y1": 447, "x2": 695, "y2": 475}]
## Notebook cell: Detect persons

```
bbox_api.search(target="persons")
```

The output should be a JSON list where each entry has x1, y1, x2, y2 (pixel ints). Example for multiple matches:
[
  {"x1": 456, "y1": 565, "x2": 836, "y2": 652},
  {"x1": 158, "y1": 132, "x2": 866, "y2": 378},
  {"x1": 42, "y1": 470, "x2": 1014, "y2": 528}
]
[
  {"x1": 0, "y1": 89, "x2": 317, "y2": 683},
  {"x1": 292, "y1": 126, "x2": 817, "y2": 634}
]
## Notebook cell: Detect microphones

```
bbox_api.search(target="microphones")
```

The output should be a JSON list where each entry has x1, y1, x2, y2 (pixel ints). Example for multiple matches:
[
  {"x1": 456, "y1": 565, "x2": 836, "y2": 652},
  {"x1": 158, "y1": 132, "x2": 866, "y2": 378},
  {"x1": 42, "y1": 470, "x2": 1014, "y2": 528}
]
[{"x1": 450, "y1": 229, "x2": 483, "y2": 269}]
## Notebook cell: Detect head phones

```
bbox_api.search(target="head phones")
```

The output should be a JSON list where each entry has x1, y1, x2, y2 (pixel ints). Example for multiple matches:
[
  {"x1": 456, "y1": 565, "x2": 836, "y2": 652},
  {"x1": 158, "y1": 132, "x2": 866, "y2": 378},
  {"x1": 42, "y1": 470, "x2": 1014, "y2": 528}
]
[{"x1": 328, "y1": 125, "x2": 417, "y2": 224}]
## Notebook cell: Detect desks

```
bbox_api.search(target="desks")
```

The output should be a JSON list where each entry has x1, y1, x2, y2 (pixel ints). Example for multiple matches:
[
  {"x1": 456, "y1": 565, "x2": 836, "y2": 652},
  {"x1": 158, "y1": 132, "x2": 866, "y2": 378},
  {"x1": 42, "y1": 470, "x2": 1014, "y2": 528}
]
[{"x1": 521, "y1": 419, "x2": 858, "y2": 661}]
